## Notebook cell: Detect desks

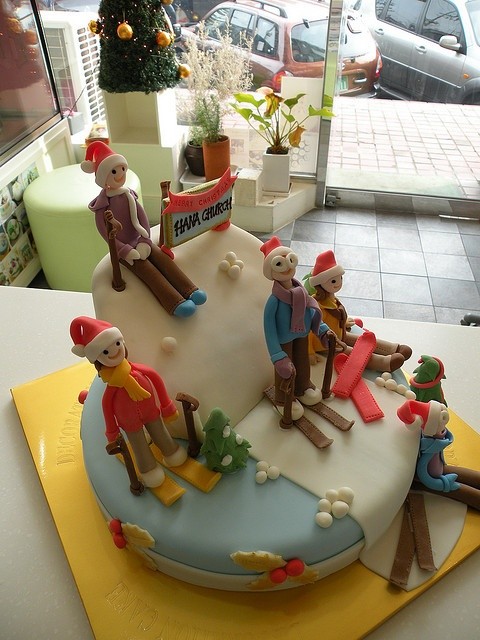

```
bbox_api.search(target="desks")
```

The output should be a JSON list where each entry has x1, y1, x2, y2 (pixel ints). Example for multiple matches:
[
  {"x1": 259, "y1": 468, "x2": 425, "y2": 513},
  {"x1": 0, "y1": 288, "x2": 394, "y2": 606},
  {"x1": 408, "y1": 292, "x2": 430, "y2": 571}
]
[{"x1": 1, "y1": 284, "x2": 478, "y2": 640}]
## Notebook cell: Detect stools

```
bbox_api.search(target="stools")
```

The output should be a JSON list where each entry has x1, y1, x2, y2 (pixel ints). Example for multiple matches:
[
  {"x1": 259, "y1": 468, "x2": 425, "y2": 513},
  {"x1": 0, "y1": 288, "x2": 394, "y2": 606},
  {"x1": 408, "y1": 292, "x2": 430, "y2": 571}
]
[{"x1": 25, "y1": 162, "x2": 142, "y2": 291}]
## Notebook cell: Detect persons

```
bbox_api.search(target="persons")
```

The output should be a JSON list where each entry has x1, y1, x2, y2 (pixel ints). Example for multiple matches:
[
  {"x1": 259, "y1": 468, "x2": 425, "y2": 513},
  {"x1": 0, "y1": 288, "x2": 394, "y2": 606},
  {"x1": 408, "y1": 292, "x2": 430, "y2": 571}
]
[
  {"x1": 260, "y1": 236, "x2": 337, "y2": 422},
  {"x1": 84, "y1": 136, "x2": 207, "y2": 318},
  {"x1": 306, "y1": 248, "x2": 412, "y2": 374},
  {"x1": 69, "y1": 315, "x2": 189, "y2": 490},
  {"x1": 408, "y1": 353, "x2": 451, "y2": 408},
  {"x1": 398, "y1": 398, "x2": 480, "y2": 517}
]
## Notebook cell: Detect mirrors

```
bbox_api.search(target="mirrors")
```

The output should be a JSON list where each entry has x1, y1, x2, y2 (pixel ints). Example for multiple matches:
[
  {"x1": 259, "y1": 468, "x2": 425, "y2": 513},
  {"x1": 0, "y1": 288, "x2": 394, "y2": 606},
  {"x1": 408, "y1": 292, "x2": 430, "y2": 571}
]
[{"x1": 0, "y1": 1, "x2": 63, "y2": 165}]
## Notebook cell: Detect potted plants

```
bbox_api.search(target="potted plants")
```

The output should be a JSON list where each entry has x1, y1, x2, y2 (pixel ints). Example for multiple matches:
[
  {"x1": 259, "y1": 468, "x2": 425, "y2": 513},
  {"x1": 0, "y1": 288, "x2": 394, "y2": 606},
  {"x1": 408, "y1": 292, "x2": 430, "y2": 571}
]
[
  {"x1": 231, "y1": 87, "x2": 335, "y2": 197},
  {"x1": 194, "y1": 96, "x2": 228, "y2": 181},
  {"x1": 184, "y1": 16, "x2": 253, "y2": 176}
]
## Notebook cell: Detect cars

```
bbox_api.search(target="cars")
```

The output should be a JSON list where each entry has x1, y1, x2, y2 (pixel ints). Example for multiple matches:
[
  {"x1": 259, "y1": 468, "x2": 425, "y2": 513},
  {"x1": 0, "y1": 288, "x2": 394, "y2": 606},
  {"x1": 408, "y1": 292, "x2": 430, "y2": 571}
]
[
  {"x1": 351, "y1": 0, "x2": 480, "y2": 104},
  {"x1": 174, "y1": 0, "x2": 382, "y2": 100}
]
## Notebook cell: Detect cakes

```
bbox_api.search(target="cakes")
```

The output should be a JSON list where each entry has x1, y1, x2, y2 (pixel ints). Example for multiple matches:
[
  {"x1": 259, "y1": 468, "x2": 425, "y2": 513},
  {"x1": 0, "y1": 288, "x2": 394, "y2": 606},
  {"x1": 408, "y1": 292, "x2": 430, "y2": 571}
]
[{"x1": 64, "y1": 139, "x2": 480, "y2": 592}]
